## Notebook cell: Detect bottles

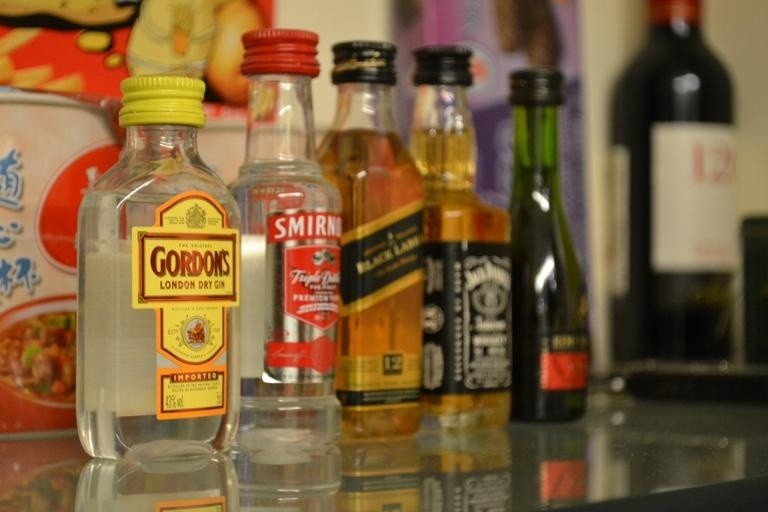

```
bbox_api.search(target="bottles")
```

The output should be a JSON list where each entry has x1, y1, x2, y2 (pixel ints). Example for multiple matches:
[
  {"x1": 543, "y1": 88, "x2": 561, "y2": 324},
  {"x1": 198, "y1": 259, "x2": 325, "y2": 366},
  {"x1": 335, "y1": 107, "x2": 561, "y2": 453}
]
[
  {"x1": 76, "y1": 466, "x2": 235, "y2": 511},
  {"x1": 236, "y1": 410, "x2": 512, "y2": 512},
  {"x1": 507, "y1": 70, "x2": 591, "y2": 417},
  {"x1": 608, "y1": 4, "x2": 751, "y2": 403},
  {"x1": 409, "y1": 42, "x2": 513, "y2": 409},
  {"x1": 515, "y1": 421, "x2": 592, "y2": 510},
  {"x1": 75, "y1": 68, "x2": 241, "y2": 460},
  {"x1": 326, "y1": 38, "x2": 430, "y2": 405},
  {"x1": 240, "y1": 26, "x2": 339, "y2": 406},
  {"x1": 602, "y1": 407, "x2": 755, "y2": 496}
]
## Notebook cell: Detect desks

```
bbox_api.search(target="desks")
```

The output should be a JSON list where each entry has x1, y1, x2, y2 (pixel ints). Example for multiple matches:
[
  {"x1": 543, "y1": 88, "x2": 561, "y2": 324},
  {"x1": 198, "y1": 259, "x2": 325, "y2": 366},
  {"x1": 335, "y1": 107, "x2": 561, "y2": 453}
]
[{"x1": 1, "y1": 392, "x2": 767, "y2": 512}]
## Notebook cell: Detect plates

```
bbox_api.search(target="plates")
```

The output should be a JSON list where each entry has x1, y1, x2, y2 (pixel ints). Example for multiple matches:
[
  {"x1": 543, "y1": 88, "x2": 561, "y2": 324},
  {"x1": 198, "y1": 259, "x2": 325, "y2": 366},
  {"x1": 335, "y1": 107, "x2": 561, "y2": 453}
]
[{"x1": 0, "y1": 292, "x2": 75, "y2": 409}]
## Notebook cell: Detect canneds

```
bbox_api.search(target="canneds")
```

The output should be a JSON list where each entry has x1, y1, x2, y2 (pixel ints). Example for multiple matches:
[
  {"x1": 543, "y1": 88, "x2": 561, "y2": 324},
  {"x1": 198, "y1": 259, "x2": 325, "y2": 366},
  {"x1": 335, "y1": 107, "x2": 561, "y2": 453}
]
[{"x1": 0, "y1": 89, "x2": 121, "y2": 441}]
